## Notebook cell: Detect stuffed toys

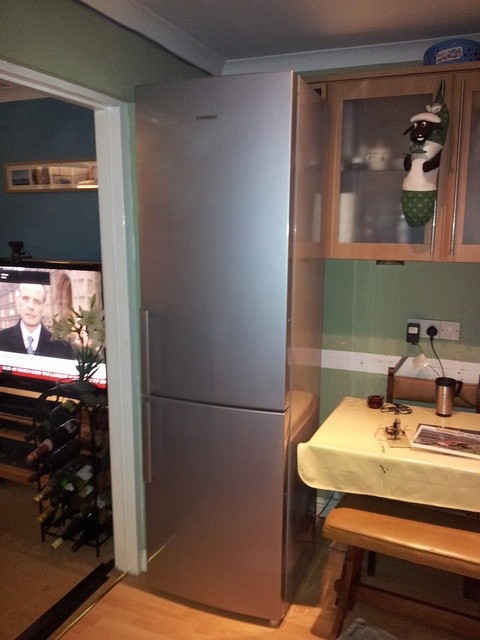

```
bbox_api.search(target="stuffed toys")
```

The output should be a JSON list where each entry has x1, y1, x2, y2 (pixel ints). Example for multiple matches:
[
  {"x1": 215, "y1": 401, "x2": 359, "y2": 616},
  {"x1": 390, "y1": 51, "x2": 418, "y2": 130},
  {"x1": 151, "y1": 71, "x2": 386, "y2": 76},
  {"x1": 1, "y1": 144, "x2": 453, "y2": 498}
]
[{"x1": 402, "y1": 80, "x2": 450, "y2": 227}]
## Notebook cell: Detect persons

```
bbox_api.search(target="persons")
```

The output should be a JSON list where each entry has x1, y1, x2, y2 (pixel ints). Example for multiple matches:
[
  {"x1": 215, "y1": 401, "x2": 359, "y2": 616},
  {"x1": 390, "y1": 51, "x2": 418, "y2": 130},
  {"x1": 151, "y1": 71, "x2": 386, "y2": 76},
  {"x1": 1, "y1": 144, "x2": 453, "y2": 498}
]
[{"x1": 0, "y1": 282, "x2": 73, "y2": 361}]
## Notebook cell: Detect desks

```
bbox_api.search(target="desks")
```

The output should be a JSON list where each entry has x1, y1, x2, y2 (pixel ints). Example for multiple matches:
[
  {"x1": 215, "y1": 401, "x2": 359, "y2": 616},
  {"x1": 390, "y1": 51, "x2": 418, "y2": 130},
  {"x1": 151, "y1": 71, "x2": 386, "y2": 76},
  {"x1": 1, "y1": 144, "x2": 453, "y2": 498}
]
[{"x1": 297, "y1": 395, "x2": 479, "y2": 505}]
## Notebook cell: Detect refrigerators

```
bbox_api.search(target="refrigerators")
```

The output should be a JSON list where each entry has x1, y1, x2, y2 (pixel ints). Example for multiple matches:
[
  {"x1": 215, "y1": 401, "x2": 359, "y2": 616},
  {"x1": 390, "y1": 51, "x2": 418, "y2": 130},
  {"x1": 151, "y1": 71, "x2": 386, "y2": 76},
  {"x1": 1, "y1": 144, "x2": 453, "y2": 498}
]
[{"x1": 132, "y1": 68, "x2": 332, "y2": 631}]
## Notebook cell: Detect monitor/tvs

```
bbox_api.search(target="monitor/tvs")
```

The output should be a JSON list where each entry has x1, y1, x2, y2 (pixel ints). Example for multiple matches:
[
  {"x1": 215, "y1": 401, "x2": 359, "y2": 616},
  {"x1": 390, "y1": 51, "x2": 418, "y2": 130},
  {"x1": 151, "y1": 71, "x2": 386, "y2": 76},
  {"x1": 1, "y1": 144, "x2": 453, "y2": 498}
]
[{"x1": 1, "y1": 257, "x2": 107, "y2": 400}]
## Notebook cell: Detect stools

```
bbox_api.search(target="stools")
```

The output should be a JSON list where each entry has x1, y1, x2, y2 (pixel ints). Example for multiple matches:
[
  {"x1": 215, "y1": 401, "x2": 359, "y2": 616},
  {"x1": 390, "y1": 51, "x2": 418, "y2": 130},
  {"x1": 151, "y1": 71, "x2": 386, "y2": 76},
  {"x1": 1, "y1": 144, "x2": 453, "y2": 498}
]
[{"x1": 312, "y1": 489, "x2": 479, "y2": 639}]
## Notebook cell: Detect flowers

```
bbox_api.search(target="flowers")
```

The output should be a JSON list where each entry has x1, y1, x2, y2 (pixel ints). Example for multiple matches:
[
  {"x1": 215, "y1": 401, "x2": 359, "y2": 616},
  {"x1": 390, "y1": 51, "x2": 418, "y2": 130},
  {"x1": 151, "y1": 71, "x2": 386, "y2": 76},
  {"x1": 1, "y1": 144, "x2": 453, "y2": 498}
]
[{"x1": 48, "y1": 293, "x2": 108, "y2": 383}]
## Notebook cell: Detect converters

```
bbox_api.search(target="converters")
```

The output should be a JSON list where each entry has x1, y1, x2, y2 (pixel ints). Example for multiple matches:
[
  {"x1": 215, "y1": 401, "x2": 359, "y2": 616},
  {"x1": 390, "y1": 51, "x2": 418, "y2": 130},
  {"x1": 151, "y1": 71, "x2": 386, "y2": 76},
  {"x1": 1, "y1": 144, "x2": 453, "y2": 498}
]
[{"x1": 405, "y1": 322, "x2": 419, "y2": 346}]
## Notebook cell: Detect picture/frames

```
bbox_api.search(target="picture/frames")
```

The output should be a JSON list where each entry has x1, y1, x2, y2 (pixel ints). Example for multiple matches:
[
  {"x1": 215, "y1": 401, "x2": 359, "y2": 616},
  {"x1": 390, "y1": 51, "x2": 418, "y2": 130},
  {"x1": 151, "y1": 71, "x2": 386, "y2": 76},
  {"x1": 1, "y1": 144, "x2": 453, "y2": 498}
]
[{"x1": 5, "y1": 160, "x2": 99, "y2": 191}]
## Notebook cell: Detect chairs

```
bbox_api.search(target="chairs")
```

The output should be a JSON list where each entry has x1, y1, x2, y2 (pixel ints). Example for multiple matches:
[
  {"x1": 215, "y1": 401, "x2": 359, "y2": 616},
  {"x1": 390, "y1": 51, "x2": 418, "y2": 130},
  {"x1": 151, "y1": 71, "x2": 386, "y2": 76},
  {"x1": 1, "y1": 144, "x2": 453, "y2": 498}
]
[{"x1": 386, "y1": 367, "x2": 479, "y2": 412}]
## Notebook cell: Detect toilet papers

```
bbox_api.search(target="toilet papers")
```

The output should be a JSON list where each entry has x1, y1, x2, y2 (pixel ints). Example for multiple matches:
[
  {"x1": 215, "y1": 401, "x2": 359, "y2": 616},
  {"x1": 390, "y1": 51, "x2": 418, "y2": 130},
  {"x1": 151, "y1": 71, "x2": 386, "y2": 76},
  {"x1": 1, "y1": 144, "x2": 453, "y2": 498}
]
[{"x1": 312, "y1": 192, "x2": 355, "y2": 244}]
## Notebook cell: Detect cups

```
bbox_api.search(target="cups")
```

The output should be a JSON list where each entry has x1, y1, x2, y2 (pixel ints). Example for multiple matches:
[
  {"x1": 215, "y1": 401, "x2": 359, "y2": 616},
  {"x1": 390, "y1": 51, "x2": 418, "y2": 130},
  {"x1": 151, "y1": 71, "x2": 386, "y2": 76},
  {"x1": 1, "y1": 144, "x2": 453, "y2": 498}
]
[
  {"x1": 435, "y1": 377, "x2": 464, "y2": 418},
  {"x1": 366, "y1": 142, "x2": 389, "y2": 171}
]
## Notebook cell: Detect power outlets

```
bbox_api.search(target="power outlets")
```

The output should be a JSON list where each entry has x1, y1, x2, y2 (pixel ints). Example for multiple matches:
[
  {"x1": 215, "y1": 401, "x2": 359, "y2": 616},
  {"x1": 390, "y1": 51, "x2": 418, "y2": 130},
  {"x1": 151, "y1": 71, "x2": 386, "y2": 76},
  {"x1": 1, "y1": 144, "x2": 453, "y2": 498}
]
[
  {"x1": 424, "y1": 319, "x2": 440, "y2": 338},
  {"x1": 440, "y1": 321, "x2": 460, "y2": 340},
  {"x1": 408, "y1": 318, "x2": 423, "y2": 339}
]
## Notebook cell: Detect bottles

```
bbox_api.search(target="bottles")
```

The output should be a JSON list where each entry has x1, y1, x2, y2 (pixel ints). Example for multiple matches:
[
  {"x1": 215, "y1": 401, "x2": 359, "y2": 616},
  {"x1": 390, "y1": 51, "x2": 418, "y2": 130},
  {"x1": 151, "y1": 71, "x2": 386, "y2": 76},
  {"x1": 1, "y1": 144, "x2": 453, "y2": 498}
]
[
  {"x1": 35, "y1": 463, "x2": 94, "y2": 526},
  {"x1": 25, "y1": 418, "x2": 78, "y2": 462},
  {"x1": 70, "y1": 504, "x2": 112, "y2": 554},
  {"x1": 23, "y1": 397, "x2": 77, "y2": 444},
  {"x1": 33, "y1": 455, "x2": 89, "y2": 502},
  {"x1": 52, "y1": 462, "x2": 99, "y2": 506},
  {"x1": 50, "y1": 495, "x2": 106, "y2": 551},
  {"x1": 53, "y1": 485, "x2": 99, "y2": 529},
  {"x1": 25, "y1": 437, "x2": 83, "y2": 486}
]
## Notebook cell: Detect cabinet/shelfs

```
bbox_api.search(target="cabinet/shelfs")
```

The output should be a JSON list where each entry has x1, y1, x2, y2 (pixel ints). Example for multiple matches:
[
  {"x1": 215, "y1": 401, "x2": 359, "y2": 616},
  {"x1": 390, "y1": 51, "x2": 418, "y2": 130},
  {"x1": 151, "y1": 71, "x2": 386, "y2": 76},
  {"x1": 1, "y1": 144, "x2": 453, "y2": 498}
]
[
  {"x1": 0, "y1": 382, "x2": 89, "y2": 486},
  {"x1": 325, "y1": 66, "x2": 476, "y2": 260},
  {"x1": 28, "y1": 385, "x2": 110, "y2": 556}
]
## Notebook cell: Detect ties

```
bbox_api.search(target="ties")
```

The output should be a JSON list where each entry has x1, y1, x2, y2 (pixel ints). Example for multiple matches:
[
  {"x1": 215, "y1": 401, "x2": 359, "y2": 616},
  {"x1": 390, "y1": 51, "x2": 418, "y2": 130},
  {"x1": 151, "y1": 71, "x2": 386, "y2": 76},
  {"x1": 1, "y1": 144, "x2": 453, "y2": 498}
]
[{"x1": 26, "y1": 337, "x2": 34, "y2": 355}]
